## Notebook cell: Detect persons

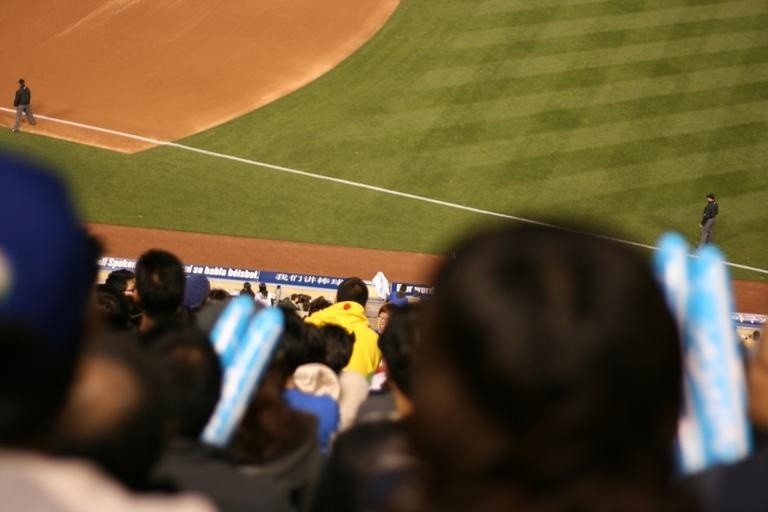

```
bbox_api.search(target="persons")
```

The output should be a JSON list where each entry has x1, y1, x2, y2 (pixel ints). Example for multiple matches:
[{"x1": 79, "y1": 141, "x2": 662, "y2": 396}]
[
  {"x1": 0, "y1": 151, "x2": 216, "y2": 512},
  {"x1": 9, "y1": 79, "x2": 35, "y2": 132},
  {"x1": 61, "y1": 221, "x2": 767, "y2": 512},
  {"x1": 700, "y1": 193, "x2": 718, "y2": 245}
]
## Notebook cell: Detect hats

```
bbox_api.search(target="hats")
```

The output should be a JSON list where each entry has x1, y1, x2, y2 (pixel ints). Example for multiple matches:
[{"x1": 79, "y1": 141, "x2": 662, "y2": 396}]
[{"x1": 0, "y1": 158, "x2": 103, "y2": 317}]
